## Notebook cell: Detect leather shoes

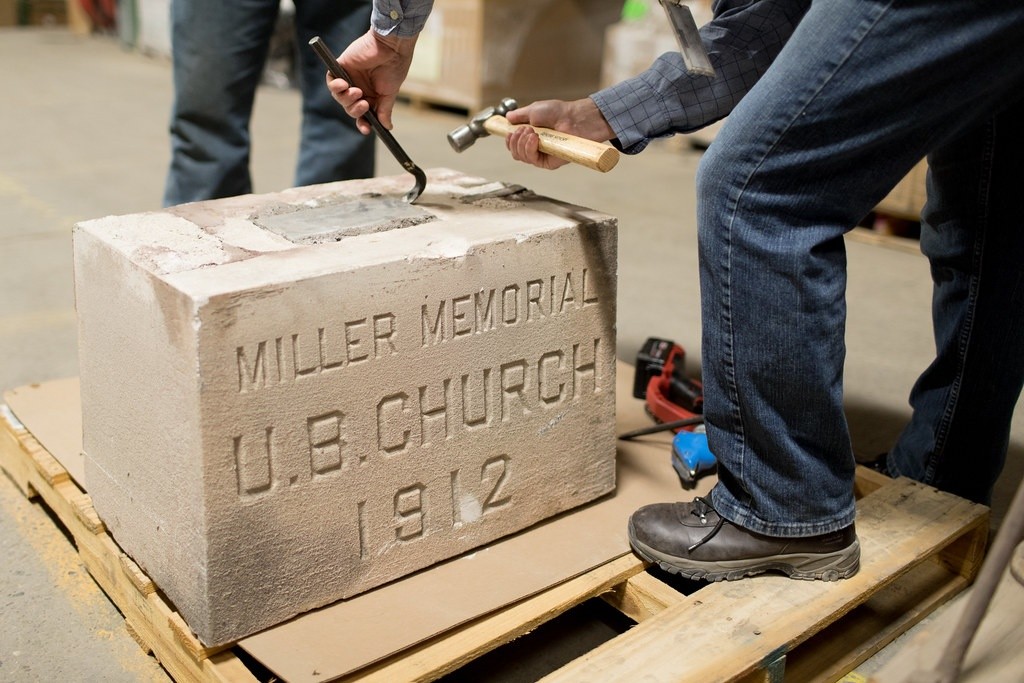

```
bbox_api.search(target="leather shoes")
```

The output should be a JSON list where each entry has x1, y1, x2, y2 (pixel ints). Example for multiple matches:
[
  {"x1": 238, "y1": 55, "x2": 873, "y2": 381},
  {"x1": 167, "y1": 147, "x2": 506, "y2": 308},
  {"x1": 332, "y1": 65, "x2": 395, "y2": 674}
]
[
  {"x1": 628, "y1": 488, "x2": 860, "y2": 582},
  {"x1": 855, "y1": 452, "x2": 893, "y2": 478}
]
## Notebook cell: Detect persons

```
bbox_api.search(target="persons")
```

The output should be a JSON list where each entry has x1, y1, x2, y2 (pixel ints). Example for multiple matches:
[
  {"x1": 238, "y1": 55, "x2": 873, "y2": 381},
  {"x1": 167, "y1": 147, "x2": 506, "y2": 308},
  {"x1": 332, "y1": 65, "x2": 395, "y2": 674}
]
[
  {"x1": 324, "y1": 0, "x2": 1024, "y2": 583},
  {"x1": 162, "y1": 0, "x2": 377, "y2": 208}
]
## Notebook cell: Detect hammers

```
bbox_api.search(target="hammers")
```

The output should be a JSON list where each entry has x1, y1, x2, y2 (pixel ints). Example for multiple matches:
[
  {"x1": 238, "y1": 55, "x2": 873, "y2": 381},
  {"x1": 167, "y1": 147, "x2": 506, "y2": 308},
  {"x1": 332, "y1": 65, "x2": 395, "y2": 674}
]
[{"x1": 446, "y1": 97, "x2": 620, "y2": 173}]
